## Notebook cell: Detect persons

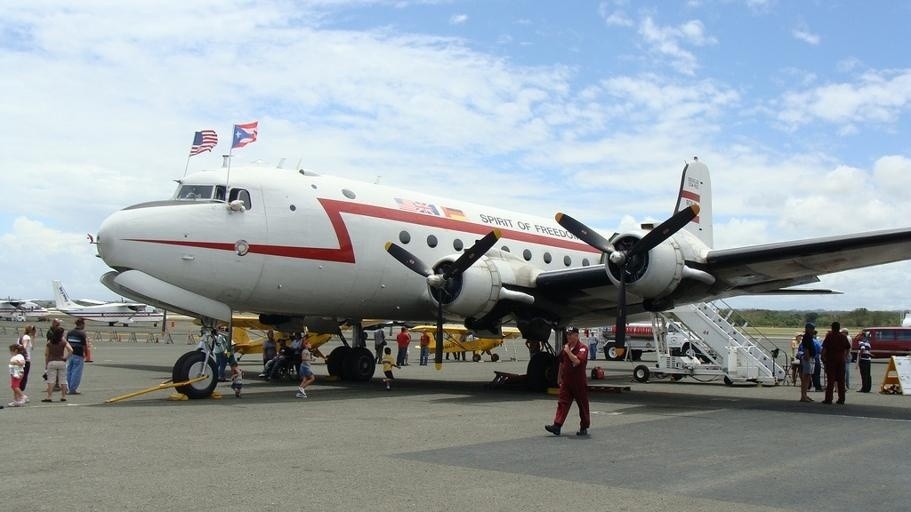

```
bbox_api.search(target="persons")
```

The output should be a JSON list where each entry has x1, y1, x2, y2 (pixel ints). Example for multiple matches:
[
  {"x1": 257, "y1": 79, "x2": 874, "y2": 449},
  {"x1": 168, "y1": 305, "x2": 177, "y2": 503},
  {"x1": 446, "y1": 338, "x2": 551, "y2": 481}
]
[
  {"x1": 46, "y1": 318, "x2": 62, "y2": 391},
  {"x1": 42, "y1": 326, "x2": 74, "y2": 402},
  {"x1": 589, "y1": 331, "x2": 599, "y2": 360},
  {"x1": 17, "y1": 325, "x2": 37, "y2": 402},
  {"x1": 396, "y1": 327, "x2": 411, "y2": 367},
  {"x1": 543, "y1": 326, "x2": 592, "y2": 435},
  {"x1": 259, "y1": 330, "x2": 316, "y2": 399},
  {"x1": 420, "y1": 332, "x2": 431, "y2": 366},
  {"x1": 458, "y1": 333, "x2": 467, "y2": 361},
  {"x1": 363, "y1": 331, "x2": 368, "y2": 348},
  {"x1": 405, "y1": 328, "x2": 412, "y2": 366},
  {"x1": 446, "y1": 333, "x2": 458, "y2": 360},
  {"x1": 381, "y1": 347, "x2": 402, "y2": 391},
  {"x1": 791, "y1": 322, "x2": 874, "y2": 404},
  {"x1": 215, "y1": 325, "x2": 231, "y2": 381},
  {"x1": 374, "y1": 328, "x2": 386, "y2": 365},
  {"x1": 229, "y1": 362, "x2": 244, "y2": 397},
  {"x1": 67, "y1": 317, "x2": 92, "y2": 394},
  {"x1": 8, "y1": 344, "x2": 30, "y2": 407}
]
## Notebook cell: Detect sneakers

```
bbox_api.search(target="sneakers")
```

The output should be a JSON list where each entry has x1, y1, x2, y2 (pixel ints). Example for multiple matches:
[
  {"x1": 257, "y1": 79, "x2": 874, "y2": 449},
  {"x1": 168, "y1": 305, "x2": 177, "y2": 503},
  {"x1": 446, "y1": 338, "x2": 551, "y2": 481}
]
[
  {"x1": 575, "y1": 428, "x2": 588, "y2": 436},
  {"x1": 545, "y1": 422, "x2": 561, "y2": 436},
  {"x1": 836, "y1": 399, "x2": 844, "y2": 404},
  {"x1": 7, "y1": 394, "x2": 30, "y2": 407},
  {"x1": 822, "y1": 400, "x2": 833, "y2": 405},
  {"x1": 296, "y1": 387, "x2": 307, "y2": 399}
]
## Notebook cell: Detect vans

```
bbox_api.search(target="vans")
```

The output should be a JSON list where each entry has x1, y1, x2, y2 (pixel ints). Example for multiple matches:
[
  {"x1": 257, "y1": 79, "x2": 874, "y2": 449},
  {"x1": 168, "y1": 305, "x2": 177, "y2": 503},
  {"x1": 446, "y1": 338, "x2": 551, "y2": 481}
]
[{"x1": 850, "y1": 327, "x2": 911, "y2": 362}]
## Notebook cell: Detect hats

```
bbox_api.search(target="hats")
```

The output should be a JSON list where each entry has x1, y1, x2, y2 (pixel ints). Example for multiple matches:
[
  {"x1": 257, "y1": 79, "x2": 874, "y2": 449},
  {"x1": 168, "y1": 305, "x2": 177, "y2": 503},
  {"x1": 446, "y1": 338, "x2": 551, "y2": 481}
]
[
  {"x1": 805, "y1": 322, "x2": 817, "y2": 328},
  {"x1": 839, "y1": 328, "x2": 848, "y2": 333},
  {"x1": 830, "y1": 322, "x2": 841, "y2": 326},
  {"x1": 565, "y1": 327, "x2": 581, "y2": 334}
]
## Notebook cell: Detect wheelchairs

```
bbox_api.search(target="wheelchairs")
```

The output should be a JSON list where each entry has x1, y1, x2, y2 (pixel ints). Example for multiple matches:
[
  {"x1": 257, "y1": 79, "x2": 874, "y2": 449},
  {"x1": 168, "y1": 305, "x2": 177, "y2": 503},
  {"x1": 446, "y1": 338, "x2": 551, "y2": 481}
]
[{"x1": 277, "y1": 353, "x2": 302, "y2": 380}]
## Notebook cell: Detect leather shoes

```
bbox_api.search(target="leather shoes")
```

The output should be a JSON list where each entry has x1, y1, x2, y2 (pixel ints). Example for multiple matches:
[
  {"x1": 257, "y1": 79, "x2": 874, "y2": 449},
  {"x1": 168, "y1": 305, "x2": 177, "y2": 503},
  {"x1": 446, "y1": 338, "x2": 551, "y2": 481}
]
[
  {"x1": 41, "y1": 399, "x2": 52, "y2": 402},
  {"x1": 61, "y1": 398, "x2": 66, "y2": 402}
]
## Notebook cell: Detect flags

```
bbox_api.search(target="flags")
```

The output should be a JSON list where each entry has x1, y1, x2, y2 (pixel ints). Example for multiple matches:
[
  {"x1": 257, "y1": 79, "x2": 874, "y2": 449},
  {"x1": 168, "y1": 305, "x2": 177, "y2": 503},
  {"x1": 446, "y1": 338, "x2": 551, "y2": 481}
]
[
  {"x1": 189, "y1": 130, "x2": 219, "y2": 157},
  {"x1": 231, "y1": 122, "x2": 258, "y2": 149}
]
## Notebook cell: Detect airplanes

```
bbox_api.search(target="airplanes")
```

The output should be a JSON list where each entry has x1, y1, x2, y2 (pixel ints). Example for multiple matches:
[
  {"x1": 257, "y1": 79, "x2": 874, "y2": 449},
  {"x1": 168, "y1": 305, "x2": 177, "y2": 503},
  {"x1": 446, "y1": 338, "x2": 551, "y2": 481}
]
[
  {"x1": 166, "y1": 312, "x2": 354, "y2": 361},
  {"x1": 0, "y1": 298, "x2": 51, "y2": 321},
  {"x1": 82, "y1": 119, "x2": 911, "y2": 398},
  {"x1": 406, "y1": 321, "x2": 523, "y2": 362},
  {"x1": 49, "y1": 279, "x2": 169, "y2": 328}
]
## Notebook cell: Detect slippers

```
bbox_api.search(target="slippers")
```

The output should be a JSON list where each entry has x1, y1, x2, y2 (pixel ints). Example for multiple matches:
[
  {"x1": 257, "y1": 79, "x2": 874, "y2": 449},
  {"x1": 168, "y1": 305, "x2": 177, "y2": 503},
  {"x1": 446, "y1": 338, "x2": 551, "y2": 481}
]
[{"x1": 800, "y1": 397, "x2": 815, "y2": 403}]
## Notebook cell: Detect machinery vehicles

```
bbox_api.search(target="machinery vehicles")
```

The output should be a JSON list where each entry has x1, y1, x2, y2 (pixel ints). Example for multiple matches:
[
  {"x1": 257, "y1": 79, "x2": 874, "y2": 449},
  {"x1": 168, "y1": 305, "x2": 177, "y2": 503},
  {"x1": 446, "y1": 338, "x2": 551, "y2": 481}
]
[
  {"x1": 603, "y1": 320, "x2": 717, "y2": 363},
  {"x1": 631, "y1": 292, "x2": 790, "y2": 384}
]
